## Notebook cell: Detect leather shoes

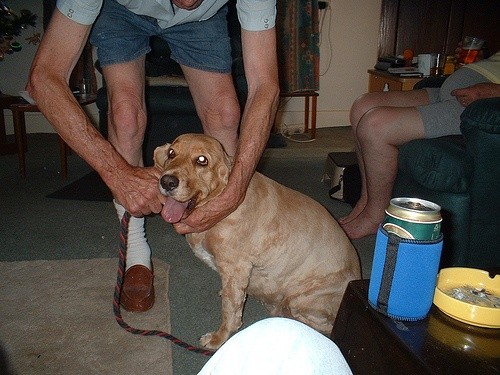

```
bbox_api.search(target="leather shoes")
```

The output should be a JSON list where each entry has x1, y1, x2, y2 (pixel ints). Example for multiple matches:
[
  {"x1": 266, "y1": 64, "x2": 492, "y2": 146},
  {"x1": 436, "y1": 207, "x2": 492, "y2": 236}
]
[{"x1": 120, "y1": 260, "x2": 154, "y2": 314}]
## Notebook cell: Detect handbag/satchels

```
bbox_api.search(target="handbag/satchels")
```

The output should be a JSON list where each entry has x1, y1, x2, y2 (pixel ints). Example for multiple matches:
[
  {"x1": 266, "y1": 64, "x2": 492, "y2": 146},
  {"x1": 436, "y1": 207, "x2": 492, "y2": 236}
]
[{"x1": 328, "y1": 165, "x2": 363, "y2": 209}]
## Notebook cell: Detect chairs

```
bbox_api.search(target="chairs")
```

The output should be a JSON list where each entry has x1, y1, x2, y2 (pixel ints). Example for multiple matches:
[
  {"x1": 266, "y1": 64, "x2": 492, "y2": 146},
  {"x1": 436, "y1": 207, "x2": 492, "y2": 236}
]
[{"x1": 11, "y1": 35, "x2": 108, "y2": 185}]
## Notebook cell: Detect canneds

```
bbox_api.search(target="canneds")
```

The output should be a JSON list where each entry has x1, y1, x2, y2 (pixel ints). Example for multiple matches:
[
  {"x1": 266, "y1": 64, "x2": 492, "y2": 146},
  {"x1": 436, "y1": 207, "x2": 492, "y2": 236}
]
[
  {"x1": 382, "y1": 197, "x2": 441, "y2": 241},
  {"x1": 444, "y1": 57, "x2": 455, "y2": 75}
]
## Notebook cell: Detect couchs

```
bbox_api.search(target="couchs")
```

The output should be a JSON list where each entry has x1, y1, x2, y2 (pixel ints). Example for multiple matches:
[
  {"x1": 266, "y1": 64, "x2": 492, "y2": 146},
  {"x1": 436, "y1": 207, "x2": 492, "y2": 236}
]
[
  {"x1": 95, "y1": 0, "x2": 246, "y2": 168},
  {"x1": 395, "y1": 74, "x2": 500, "y2": 269}
]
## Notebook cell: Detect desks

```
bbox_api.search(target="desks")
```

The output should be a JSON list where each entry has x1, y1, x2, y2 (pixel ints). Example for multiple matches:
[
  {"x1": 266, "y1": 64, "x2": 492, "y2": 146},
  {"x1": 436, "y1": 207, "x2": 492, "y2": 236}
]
[{"x1": 333, "y1": 279, "x2": 500, "y2": 375}]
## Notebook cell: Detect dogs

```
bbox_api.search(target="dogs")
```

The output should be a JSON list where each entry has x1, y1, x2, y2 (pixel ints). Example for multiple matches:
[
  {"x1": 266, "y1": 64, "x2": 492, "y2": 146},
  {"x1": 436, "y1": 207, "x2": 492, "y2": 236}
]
[{"x1": 152, "y1": 133, "x2": 362, "y2": 352}]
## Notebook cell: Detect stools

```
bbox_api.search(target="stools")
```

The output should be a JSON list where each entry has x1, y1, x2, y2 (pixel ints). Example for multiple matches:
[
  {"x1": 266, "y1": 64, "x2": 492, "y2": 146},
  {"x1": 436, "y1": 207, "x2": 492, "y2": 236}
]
[{"x1": 280, "y1": 91, "x2": 319, "y2": 139}]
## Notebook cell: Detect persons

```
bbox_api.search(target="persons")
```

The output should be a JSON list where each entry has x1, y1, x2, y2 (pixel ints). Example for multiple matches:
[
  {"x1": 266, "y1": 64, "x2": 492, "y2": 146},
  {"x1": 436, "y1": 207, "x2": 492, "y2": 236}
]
[
  {"x1": 336, "y1": 50, "x2": 500, "y2": 240},
  {"x1": 24, "y1": 0, "x2": 280, "y2": 314}
]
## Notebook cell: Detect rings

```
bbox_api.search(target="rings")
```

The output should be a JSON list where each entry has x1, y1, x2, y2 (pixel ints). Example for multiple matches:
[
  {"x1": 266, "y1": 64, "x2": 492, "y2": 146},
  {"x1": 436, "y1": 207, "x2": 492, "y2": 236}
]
[{"x1": 463, "y1": 100, "x2": 464, "y2": 102}]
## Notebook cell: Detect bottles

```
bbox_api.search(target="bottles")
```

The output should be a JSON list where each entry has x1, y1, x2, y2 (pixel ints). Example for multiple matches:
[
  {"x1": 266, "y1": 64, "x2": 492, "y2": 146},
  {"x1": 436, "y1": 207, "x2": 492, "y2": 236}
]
[{"x1": 443, "y1": 55, "x2": 457, "y2": 76}]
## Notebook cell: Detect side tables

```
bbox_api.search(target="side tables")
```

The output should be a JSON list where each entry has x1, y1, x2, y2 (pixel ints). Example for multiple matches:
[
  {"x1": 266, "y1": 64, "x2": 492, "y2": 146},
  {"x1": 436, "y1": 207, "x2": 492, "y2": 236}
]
[{"x1": 367, "y1": 69, "x2": 426, "y2": 94}]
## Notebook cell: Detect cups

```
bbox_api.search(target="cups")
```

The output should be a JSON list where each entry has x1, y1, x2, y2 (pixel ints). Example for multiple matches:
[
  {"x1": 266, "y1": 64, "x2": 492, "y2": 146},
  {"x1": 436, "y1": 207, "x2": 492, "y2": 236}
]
[
  {"x1": 459, "y1": 36, "x2": 484, "y2": 64},
  {"x1": 417, "y1": 53, "x2": 432, "y2": 76},
  {"x1": 430, "y1": 49, "x2": 444, "y2": 77}
]
